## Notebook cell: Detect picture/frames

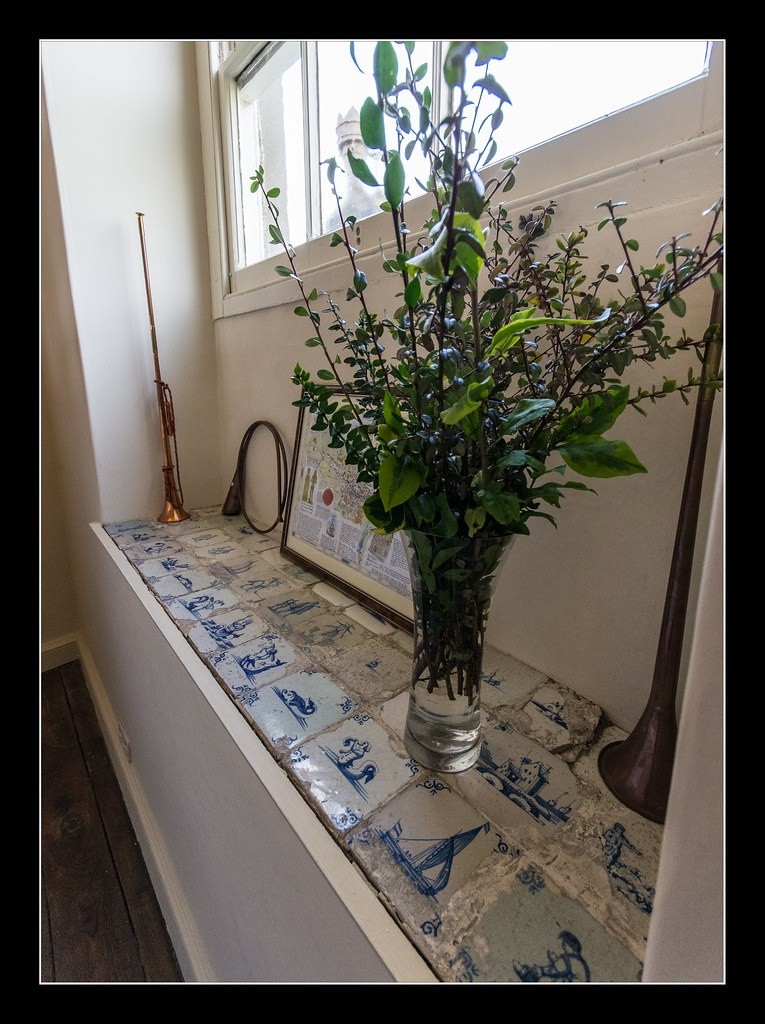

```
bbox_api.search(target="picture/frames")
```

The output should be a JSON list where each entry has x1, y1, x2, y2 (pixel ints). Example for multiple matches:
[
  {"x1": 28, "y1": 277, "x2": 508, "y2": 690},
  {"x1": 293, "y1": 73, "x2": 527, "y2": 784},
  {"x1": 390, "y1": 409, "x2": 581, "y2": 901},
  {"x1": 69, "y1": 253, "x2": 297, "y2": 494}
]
[{"x1": 280, "y1": 383, "x2": 415, "y2": 638}]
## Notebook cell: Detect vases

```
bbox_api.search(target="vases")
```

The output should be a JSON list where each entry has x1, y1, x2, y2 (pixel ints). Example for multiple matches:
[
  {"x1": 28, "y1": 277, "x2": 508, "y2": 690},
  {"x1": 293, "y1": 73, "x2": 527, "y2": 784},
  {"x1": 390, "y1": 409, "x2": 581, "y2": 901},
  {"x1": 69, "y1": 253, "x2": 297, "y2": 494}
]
[{"x1": 400, "y1": 529, "x2": 516, "y2": 773}]
